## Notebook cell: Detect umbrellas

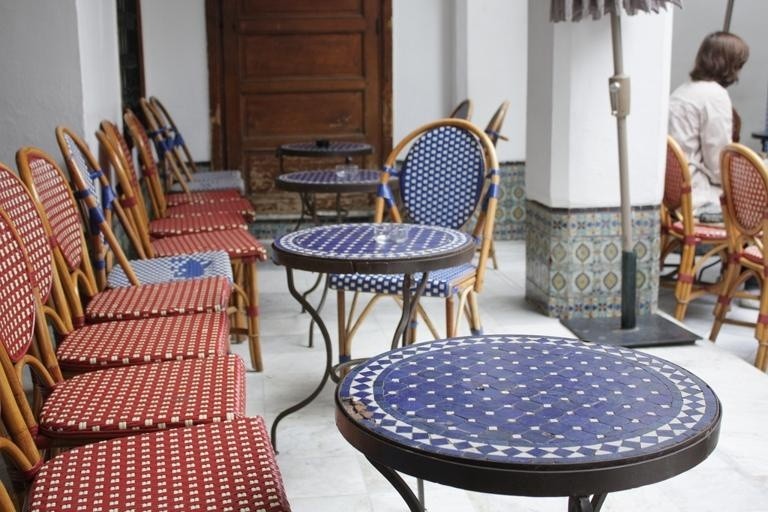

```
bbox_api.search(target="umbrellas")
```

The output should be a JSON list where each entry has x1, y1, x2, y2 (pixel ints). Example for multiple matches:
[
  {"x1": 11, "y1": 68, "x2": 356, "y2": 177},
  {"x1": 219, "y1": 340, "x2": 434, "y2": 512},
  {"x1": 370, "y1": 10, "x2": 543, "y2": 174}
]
[{"x1": 549, "y1": 0, "x2": 685, "y2": 252}]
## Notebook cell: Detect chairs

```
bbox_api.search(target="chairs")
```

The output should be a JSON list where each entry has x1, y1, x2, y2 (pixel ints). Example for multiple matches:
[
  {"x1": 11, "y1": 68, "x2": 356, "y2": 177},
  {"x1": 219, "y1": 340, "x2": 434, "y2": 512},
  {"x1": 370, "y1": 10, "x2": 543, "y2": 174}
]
[{"x1": 657, "y1": 135, "x2": 768, "y2": 372}]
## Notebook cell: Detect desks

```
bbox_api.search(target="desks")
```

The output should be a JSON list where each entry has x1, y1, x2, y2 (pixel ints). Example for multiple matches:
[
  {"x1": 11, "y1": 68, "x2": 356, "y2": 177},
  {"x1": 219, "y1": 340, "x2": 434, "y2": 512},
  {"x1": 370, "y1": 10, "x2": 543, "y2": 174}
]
[{"x1": 331, "y1": 334, "x2": 724, "y2": 512}]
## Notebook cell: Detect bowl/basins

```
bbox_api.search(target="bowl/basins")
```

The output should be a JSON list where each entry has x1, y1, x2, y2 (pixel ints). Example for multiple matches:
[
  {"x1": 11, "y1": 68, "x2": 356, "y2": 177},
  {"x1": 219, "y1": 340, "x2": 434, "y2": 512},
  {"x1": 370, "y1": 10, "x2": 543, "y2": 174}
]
[
  {"x1": 374, "y1": 225, "x2": 408, "y2": 243},
  {"x1": 335, "y1": 164, "x2": 359, "y2": 180},
  {"x1": 317, "y1": 140, "x2": 329, "y2": 147}
]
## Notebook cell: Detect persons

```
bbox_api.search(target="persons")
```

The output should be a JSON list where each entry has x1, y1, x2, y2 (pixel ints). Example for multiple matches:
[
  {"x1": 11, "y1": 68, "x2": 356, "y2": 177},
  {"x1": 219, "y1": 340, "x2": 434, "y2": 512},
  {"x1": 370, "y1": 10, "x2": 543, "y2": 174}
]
[{"x1": 666, "y1": 31, "x2": 763, "y2": 290}]
